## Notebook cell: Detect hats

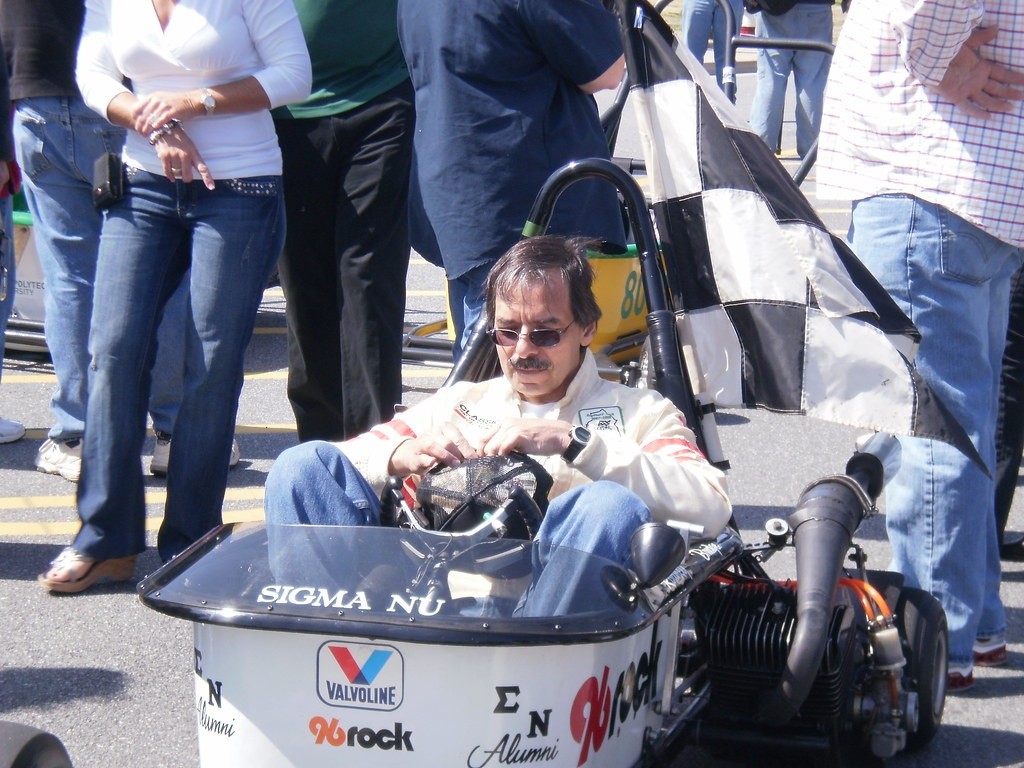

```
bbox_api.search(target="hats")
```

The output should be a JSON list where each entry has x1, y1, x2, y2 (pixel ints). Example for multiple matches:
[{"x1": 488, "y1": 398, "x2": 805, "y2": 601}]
[{"x1": 417, "y1": 448, "x2": 554, "y2": 543}]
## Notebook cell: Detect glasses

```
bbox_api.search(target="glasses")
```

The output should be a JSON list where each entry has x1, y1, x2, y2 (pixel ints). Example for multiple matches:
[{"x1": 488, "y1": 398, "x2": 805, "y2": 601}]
[{"x1": 484, "y1": 315, "x2": 579, "y2": 347}]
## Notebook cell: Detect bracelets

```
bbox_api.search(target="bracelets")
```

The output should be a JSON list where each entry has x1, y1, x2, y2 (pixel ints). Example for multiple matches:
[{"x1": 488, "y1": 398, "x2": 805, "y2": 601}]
[{"x1": 149, "y1": 120, "x2": 179, "y2": 145}]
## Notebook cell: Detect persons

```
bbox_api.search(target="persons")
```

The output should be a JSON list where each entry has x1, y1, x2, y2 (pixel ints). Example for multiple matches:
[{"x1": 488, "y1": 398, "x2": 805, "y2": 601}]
[
  {"x1": 400, "y1": 0, "x2": 626, "y2": 367},
  {"x1": 269, "y1": 0, "x2": 416, "y2": 445},
  {"x1": 994, "y1": 260, "x2": 1024, "y2": 556},
  {"x1": 38, "y1": 0, "x2": 313, "y2": 594},
  {"x1": 0, "y1": 0, "x2": 239, "y2": 482},
  {"x1": 0, "y1": 39, "x2": 26, "y2": 444},
  {"x1": 814, "y1": 0, "x2": 1024, "y2": 695},
  {"x1": 682, "y1": 0, "x2": 744, "y2": 107},
  {"x1": 742, "y1": 0, "x2": 852, "y2": 158},
  {"x1": 266, "y1": 233, "x2": 731, "y2": 621}
]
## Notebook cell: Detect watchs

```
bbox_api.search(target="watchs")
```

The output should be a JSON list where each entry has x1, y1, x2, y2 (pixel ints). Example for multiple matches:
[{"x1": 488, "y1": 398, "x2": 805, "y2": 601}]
[
  {"x1": 200, "y1": 87, "x2": 216, "y2": 117},
  {"x1": 560, "y1": 425, "x2": 591, "y2": 464}
]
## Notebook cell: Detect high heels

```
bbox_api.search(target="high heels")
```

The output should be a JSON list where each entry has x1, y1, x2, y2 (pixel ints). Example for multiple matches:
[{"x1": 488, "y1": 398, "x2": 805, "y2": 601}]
[{"x1": 39, "y1": 546, "x2": 138, "y2": 595}]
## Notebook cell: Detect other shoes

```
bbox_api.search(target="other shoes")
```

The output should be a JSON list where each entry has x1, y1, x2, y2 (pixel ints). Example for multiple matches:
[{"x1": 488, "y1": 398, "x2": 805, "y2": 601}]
[
  {"x1": 971, "y1": 638, "x2": 1009, "y2": 667},
  {"x1": 946, "y1": 664, "x2": 976, "y2": 694}
]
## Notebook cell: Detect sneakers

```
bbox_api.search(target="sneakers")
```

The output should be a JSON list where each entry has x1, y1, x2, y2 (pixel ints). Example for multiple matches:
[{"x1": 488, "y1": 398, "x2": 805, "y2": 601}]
[
  {"x1": 151, "y1": 433, "x2": 240, "y2": 474},
  {"x1": 0, "y1": 417, "x2": 26, "y2": 443},
  {"x1": 35, "y1": 433, "x2": 84, "y2": 482}
]
